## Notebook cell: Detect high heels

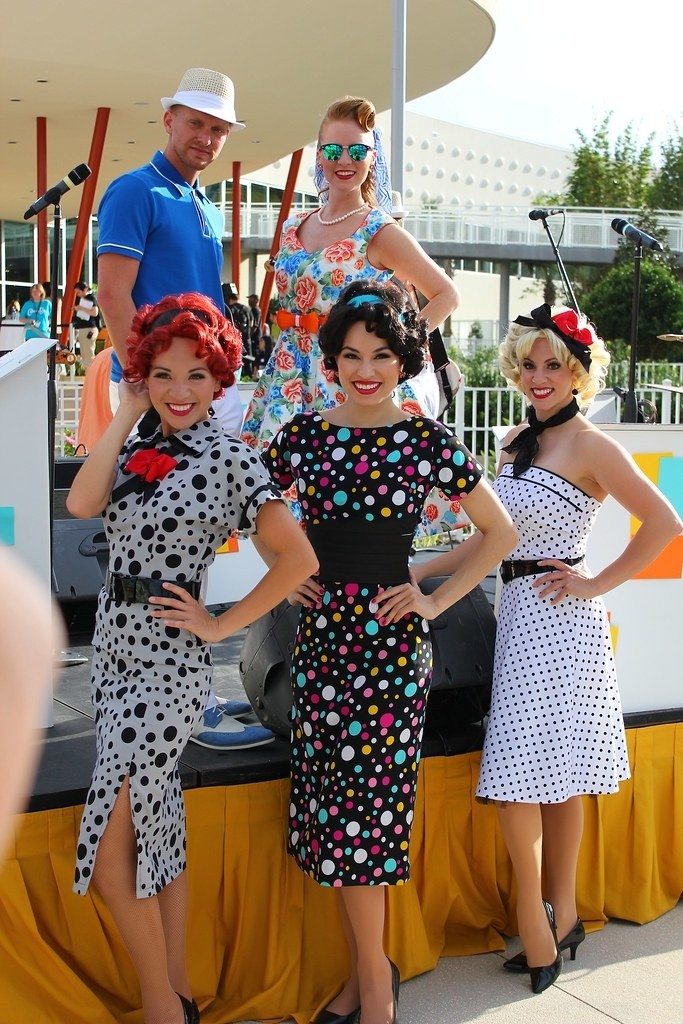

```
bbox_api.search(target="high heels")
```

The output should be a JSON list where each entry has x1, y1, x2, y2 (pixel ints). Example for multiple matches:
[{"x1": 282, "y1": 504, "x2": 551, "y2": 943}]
[
  {"x1": 503, "y1": 917, "x2": 585, "y2": 973},
  {"x1": 312, "y1": 988, "x2": 361, "y2": 1024},
  {"x1": 530, "y1": 898, "x2": 564, "y2": 994},
  {"x1": 355, "y1": 955, "x2": 401, "y2": 1024}
]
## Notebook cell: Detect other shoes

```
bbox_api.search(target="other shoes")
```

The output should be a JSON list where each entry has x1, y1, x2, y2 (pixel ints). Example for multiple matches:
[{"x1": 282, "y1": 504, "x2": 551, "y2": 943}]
[{"x1": 175, "y1": 991, "x2": 200, "y2": 1024}]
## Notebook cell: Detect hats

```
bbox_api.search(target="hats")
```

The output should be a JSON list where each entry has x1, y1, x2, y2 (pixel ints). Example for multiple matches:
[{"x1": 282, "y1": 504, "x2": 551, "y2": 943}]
[
  {"x1": 160, "y1": 68, "x2": 246, "y2": 132},
  {"x1": 246, "y1": 294, "x2": 258, "y2": 299},
  {"x1": 74, "y1": 282, "x2": 92, "y2": 292}
]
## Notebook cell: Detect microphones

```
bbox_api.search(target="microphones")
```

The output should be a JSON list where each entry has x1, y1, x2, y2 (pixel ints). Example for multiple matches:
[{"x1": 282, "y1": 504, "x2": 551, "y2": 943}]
[
  {"x1": 529, "y1": 209, "x2": 564, "y2": 221},
  {"x1": 23, "y1": 163, "x2": 91, "y2": 220},
  {"x1": 611, "y1": 218, "x2": 663, "y2": 252}
]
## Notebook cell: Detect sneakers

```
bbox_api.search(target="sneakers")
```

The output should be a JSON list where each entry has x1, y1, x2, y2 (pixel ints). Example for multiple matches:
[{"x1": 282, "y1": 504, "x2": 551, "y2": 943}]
[
  {"x1": 210, "y1": 695, "x2": 254, "y2": 719},
  {"x1": 189, "y1": 704, "x2": 275, "y2": 750}
]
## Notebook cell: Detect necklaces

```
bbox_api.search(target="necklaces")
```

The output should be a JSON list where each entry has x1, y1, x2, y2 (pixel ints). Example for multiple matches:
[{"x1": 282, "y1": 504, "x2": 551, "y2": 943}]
[{"x1": 317, "y1": 200, "x2": 369, "y2": 225}]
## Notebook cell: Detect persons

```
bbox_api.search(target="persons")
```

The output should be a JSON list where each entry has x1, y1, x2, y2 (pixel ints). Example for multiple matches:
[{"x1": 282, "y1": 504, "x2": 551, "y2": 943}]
[
  {"x1": 18, "y1": 282, "x2": 62, "y2": 379},
  {"x1": 238, "y1": 280, "x2": 520, "y2": 1024},
  {"x1": 0, "y1": 536, "x2": 63, "y2": 875},
  {"x1": 95, "y1": 68, "x2": 246, "y2": 753},
  {"x1": 409, "y1": 303, "x2": 683, "y2": 994},
  {"x1": 238, "y1": 97, "x2": 480, "y2": 623},
  {"x1": 222, "y1": 292, "x2": 281, "y2": 383},
  {"x1": 71, "y1": 281, "x2": 102, "y2": 376},
  {"x1": 65, "y1": 291, "x2": 320, "y2": 1024}
]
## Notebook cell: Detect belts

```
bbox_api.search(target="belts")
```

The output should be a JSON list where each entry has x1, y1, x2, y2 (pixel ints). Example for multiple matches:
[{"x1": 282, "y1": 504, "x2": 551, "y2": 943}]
[
  {"x1": 499, "y1": 555, "x2": 582, "y2": 584},
  {"x1": 105, "y1": 569, "x2": 203, "y2": 609}
]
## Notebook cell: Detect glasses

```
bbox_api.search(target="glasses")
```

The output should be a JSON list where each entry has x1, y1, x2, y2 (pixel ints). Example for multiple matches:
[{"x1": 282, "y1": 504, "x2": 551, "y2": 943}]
[{"x1": 319, "y1": 143, "x2": 374, "y2": 162}]
